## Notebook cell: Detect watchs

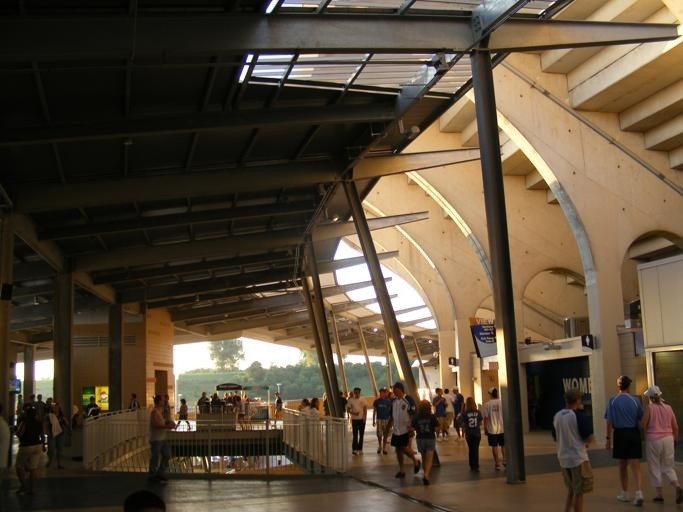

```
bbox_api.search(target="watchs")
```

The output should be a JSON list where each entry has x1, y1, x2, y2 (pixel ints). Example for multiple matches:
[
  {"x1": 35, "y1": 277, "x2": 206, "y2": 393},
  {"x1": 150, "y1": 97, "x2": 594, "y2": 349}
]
[{"x1": 605, "y1": 436, "x2": 611, "y2": 441}]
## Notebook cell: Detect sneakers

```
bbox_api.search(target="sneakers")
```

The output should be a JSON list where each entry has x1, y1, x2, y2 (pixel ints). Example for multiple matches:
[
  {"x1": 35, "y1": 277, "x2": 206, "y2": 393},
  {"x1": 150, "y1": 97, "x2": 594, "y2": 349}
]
[
  {"x1": 652, "y1": 494, "x2": 663, "y2": 502},
  {"x1": 616, "y1": 493, "x2": 631, "y2": 502},
  {"x1": 675, "y1": 486, "x2": 683, "y2": 507},
  {"x1": 393, "y1": 470, "x2": 408, "y2": 481},
  {"x1": 655, "y1": 391, "x2": 658, "y2": 392},
  {"x1": 414, "y1": 459, "x2": 422, "y2": 474},
  {"x1": 494, "y1": 463, "x2": 500, "y2": 471},
  {"x1": 631, "y1": 494, "x2": 644, "y2": 506}
]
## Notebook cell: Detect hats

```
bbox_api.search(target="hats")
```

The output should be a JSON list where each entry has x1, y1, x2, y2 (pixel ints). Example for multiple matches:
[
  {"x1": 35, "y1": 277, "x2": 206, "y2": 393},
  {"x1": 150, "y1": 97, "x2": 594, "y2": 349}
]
[
  {"x1": 450, "y1": 385, "x2": 460, "y2": 390},
  {"x1": 616, "y1": 375, "x2": 633, "y2": 387},
  {"x1": 378, "y1": 387, "x2": 387, "y2": 393},
  {"x1": 274, "y1": 390, "x2": 279, "y2": 395},
  {"x1": 563, "y1": 387, "x2": 586, "y2": 403},
  {"x1": 486, "y1": 386, "x2": 497, "y2": 394},
  {"x1": 389, "y1": 381, "x2": 403, "y2": 392},
  {"x1": 644, "y1": 385, "x2": 664, "y2": 399}
]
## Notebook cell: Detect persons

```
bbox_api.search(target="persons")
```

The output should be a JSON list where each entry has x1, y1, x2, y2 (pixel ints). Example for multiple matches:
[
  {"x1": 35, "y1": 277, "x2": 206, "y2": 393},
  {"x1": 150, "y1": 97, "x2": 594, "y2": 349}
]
[
  {"x1": 451, "y1": 385, "x2": 465, "y2": 442},
  {"x1": 348, "y1": 391, "x2": 354, "y2": 422},
  {"x1": 71, "y1": 401, "x2": 79, "y2": 416},
  {"x1": 443, "y1": 389, "x2": 455, "y2": 434},
  {"x1": 44, "y1": 402, "x2": 69, "y2": 470},
  {"x1": 175, "y1": 399, "x2": 191, "y2": 431},
  {"x1": 127, "y1": 393, "x2": 140, "y2": 412},
  {"x1": 340, "y1": 391, "x2": 347, "y2": 418},
  {"x1": 163, "y1": 395, "x2": 175, "y2": 420},
  {"x1": 432, "y1": 389, "x2": 448, "y2": 442},
  {"x1": 86, "y1": 396, "x2": 99, "y2": 417},
  {"x1": 409, "y1": 400, "x2": 440, "y2": 486},
  {"x1": 372, "y1": 389, "x2": 392, "y2": 455},
  {"x1": 271, "y1": 391, "x2": 283, "y2": 426},
  {"x1": 0, "y1": 414, "x2": 11, "y2": 501},
  {"x1": 640, "y1": 385, "x2": 683, "y2": 504},
  {"x1": 15, "y1": 394, "x2": 24, "y2": 419},
  {"x1": 298, "y1": 398, "x2": 310, "y2": 416},
  {"x1": 603, "y1": 376, "x2": 644, "y2": 507},
  {"x1": 44, "y1": 397, "x2": 53, "y2": 414},
  {"x1": 306, "y1": 397, "x2": 321, "y2": 419},
  {"x1": 197, "y1": 390, "x2": 249, "y2": 431},
  {"x1": 145, "y1": 395, "x2": 176, "y2": 484},
  {"x1": 13, "y1": 405, "x2": 46, "y2": 497},
  {"x1": 385, "y1": 383, "x2": 420, "y2": 479},
  {"x1": 482, "y1": 386, "x2": 506, "y2": 472},
  {"x1": 323, "y1": 398, "x2": 330, "y2": 417},
  {"x1": 461, "y1": 397, "x2": 483, "y2": 472},
  {"x1": 28, "y1": 394, "x2": 36, "y2": 406},
  {"x1": 551, "y1": 388, "x2": 594, "y2": 512},
  {"x1": 70, "y1": 407, "x2": 83, "y2": 462},
  {"x1": 35, "y1": 394, "x2": 46, "y2": 422},
  {"x1": 347, "y1": 387, "x2": 368, "y2": 455}
]
